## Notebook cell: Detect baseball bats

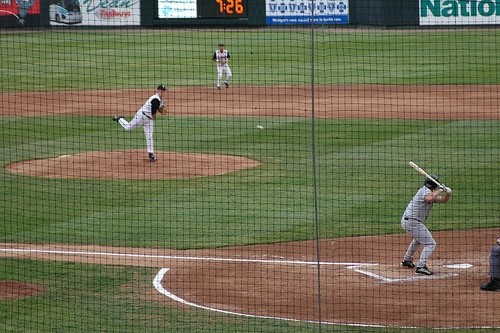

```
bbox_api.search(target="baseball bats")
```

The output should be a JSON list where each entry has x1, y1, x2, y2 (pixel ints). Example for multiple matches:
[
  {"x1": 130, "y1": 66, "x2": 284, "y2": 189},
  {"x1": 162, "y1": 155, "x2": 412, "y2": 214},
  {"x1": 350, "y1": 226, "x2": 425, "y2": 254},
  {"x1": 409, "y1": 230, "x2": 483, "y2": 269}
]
[{"x1": 409, "y1": 162, "x2": 444, "y2": 192}]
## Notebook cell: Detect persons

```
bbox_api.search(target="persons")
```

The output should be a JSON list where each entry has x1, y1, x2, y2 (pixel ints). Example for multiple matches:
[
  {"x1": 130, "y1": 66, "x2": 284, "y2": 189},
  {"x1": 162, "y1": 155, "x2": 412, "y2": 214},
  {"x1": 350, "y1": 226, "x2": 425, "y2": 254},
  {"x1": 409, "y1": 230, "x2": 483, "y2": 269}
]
[
  {"x1": 211, "y1": 43, "x2": 232, "y2": 91},
  {"x1": 401, "y1": 174, "x2": 452, "y2": 276},
  {"x1": 112, "y1": 85, "x2": 168, "y2": 159},
  {"x1": 480, "y1": 237, "x2": 500, "y2": 291}
]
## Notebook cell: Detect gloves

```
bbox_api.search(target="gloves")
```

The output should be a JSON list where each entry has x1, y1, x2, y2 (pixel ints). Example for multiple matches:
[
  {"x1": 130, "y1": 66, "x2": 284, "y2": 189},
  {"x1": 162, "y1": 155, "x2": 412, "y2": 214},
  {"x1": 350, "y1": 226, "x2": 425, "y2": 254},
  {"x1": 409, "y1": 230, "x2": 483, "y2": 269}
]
[{"x1": 437, "y1": 183, "x2": 452, "y2": 196}]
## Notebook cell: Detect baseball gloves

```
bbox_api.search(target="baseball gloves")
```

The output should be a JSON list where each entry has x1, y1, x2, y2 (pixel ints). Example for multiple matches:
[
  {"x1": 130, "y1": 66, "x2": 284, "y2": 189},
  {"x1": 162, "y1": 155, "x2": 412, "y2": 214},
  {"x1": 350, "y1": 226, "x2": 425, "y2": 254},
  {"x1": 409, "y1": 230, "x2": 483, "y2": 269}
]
[{"x1": 160, "y1": 105, "x2": 168, "y2": 115}]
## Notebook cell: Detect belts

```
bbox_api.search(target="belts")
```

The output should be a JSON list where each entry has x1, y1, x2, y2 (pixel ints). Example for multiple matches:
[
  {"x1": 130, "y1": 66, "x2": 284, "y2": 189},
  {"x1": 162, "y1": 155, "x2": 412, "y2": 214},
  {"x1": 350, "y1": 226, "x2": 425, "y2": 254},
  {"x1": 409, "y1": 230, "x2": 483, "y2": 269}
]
[
  {"x1": 404, "y1": 218, "x2": 419, "y2": 222},
  {"x1": 142, "y1": 112, "x2": 150, "y2": 118}
]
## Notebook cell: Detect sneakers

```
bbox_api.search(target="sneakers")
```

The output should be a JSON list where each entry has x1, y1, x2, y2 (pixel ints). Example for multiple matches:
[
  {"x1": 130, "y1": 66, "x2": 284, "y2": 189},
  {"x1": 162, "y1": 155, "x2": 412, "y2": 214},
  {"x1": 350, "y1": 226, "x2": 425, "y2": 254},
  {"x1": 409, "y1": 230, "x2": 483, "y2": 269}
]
[
  {"x1": 415, "y1": 264, "x2": 433, "y2": 275},
  {"x1": 402, "y1": 260, "x2": 415, "y2": 268}
]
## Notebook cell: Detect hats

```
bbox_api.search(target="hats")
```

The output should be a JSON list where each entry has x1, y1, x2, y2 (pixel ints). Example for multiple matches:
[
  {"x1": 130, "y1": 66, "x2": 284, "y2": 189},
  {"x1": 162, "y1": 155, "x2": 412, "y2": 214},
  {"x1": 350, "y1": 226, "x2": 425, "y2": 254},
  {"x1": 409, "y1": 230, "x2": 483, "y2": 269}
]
[
  {"x1": 218, "y1": 43, "x2": 225, "y2": 46},
  {"x1": 157, "y1": 84, "x2": 167, "y2": 90}
]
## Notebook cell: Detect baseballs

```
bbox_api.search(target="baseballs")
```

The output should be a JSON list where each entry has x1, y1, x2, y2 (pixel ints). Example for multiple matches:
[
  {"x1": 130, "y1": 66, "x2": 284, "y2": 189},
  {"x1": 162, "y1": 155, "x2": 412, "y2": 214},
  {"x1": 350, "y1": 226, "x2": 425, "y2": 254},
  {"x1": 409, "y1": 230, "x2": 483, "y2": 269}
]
[{"x1": 256, "y1": 124, "x2": 262, "y2": 129}]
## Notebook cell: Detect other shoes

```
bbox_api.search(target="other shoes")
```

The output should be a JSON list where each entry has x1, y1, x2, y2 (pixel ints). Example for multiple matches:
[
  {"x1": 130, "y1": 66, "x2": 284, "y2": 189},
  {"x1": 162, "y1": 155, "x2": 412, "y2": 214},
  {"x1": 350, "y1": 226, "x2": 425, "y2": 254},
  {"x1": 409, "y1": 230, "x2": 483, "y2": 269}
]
[
  {"x1": 149, "y1": 153, "x2": 154, "y2": 158},
  {"x1": 480, "y1": 279, "x2": 500, "y2": 291},
  {"x1": 224, "y1": 82, "x2": 228, "y2": 86},
  {"x1": 112, "y1": 115, "x2": 124, "y2": 122},
  {"x1": 217, "y1": 87, "x2": 220, "y2": 90}
]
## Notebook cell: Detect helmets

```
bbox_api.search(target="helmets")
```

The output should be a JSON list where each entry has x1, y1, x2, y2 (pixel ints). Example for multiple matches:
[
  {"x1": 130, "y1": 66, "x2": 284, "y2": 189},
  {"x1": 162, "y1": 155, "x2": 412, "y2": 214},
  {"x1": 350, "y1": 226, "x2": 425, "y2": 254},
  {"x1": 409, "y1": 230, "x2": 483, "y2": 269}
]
[{"x1": 425, "y1": 174, "x2": 439, "y2": 189}]
